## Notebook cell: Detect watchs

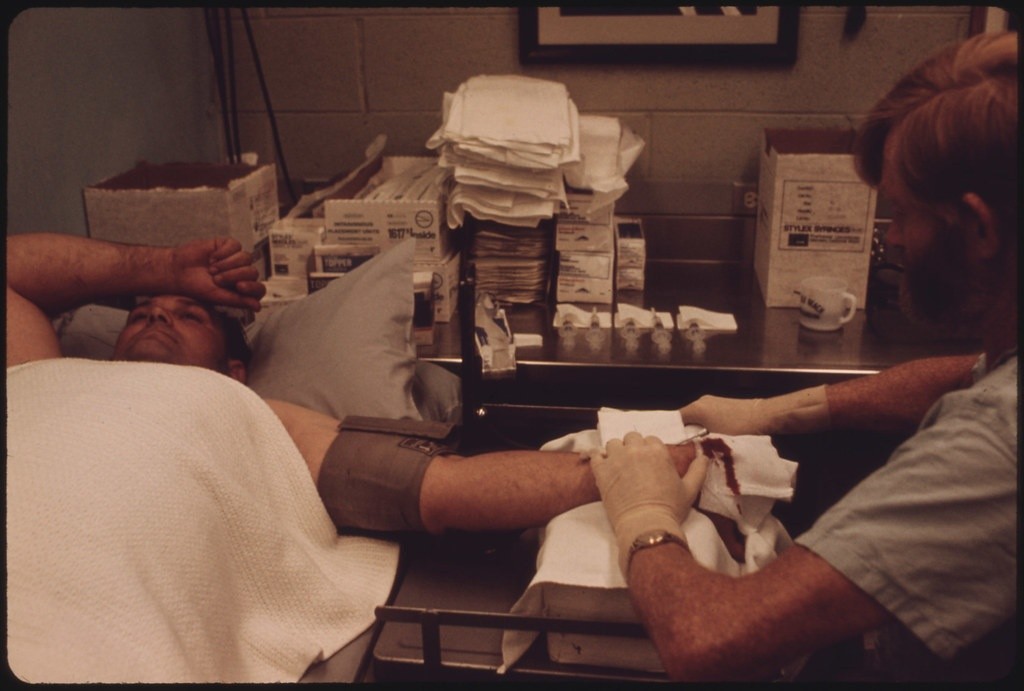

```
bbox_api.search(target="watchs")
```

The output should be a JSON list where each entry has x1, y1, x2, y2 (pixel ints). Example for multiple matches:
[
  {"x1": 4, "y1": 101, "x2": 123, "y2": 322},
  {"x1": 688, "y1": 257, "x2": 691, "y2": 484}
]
[{"x1": 628, "y1": 529, "x2": 690, "y2": 580}]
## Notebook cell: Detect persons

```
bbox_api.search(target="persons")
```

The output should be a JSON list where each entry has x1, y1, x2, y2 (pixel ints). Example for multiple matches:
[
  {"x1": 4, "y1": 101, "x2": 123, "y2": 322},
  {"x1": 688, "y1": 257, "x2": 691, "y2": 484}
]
[
  {"x1": 586, "y1": 25, "x2": 1022, "y2": 685},
  {"x1": 1, "y1": 232, "x2": 740, "y2": 689}
]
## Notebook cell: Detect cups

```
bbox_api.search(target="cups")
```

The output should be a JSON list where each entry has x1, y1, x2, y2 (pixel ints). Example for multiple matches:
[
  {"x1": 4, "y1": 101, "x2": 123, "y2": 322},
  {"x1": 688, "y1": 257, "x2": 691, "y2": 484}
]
[{"x1": 797, "y1": 276, "x2": 857, "y2": 331}]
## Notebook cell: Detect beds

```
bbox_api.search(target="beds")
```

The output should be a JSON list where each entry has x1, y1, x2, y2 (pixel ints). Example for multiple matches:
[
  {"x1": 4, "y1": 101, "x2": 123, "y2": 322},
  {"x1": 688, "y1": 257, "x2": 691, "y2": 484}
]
[{"x1": 7, "y1": 360, "x2": 464, "y2": 682}]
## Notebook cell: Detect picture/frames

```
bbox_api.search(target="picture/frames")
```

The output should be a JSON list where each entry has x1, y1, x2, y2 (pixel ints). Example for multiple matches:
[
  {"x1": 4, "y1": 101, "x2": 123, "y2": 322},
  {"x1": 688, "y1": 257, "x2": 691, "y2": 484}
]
[{"x1": 518, "y1": 0, "x2": 800, "y2": 67}]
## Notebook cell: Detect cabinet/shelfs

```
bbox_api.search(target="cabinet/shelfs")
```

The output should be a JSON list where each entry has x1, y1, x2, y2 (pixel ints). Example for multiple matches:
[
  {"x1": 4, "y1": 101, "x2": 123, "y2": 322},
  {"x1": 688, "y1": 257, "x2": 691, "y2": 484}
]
[
  {"x1": 471, "y1": 262, "x2": 982, "y2": 546},
  {"x1": 57, "y1": 202, "x2": 466, "y2": 457}
]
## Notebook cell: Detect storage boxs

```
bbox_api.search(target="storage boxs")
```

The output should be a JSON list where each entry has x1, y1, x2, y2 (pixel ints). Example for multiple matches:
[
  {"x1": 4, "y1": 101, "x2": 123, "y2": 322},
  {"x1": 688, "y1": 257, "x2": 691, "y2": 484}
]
[
  {"x1": 555, "y1": 192, "x2": 647, "y2": 307},
  {"x1": 753, "y1": 129, "x2": 881, "y2": 310},
  {"x1": 84, "y1": 159, "x2": 463, "y2": 345}
]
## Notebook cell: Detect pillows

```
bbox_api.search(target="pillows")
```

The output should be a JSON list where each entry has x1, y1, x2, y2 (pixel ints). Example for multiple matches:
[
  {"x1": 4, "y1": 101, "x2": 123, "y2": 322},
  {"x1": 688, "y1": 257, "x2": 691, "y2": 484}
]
[{"x1": 61, "y1": 238, "x2": 423, "y2": 424}]
